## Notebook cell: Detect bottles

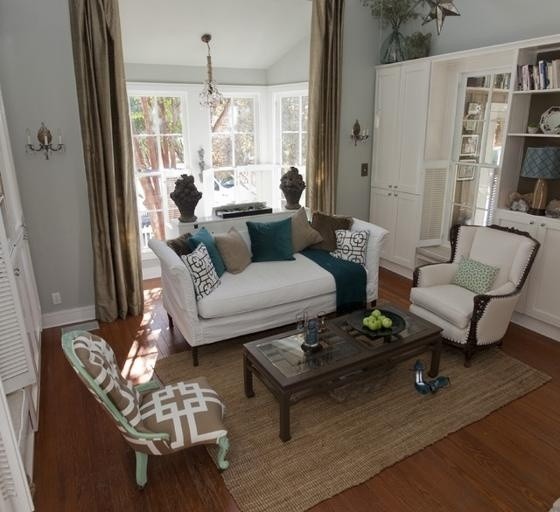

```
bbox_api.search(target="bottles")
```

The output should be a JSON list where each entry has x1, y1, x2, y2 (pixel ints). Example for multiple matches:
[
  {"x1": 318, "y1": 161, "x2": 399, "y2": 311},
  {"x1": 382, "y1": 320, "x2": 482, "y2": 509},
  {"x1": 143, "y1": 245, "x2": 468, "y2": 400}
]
[{"x1": 532, "y1": 175, "x2": 549, "y2": 209}]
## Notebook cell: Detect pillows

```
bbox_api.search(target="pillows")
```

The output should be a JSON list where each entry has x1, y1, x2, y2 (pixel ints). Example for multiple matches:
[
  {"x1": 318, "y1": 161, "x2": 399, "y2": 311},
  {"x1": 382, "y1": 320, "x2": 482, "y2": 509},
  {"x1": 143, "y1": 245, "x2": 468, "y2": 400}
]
[
  {"x1": 451, "y1": 254, "x2": 500, "y2": 295},
  {"x1": 188, "y1": 226, "x2": 226, "y2": 279},
  {"x1": 180, "y1": 240, "x2": 221, "y2": 301},
  {"x1": 287, "y1": 206, "x2": 324, "y2": 255},
  {"x1": 328, "y1": 228, "x2": 370, "y2": 265},
  {"x1": 167, "y1": 232, "x2": 198, "y2": 256},
  {"x1": 244, "y1": 217, "x2": 297, "y2": 262},
  {"x1": 308, "y1": 210, "x2": 354, "y2": 253},
  {"x1": 213, "y1": 223, "x2": 252, "y2": 276}
]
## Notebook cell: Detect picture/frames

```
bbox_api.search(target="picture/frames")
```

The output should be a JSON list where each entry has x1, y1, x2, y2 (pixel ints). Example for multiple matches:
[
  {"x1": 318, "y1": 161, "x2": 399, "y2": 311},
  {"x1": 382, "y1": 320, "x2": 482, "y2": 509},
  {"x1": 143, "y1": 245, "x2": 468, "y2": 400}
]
[
  {"x1": 457, "y1": 159, "x2": 477, "y2": 182},
  {"x1": 459, "y1": 132, "x2": 480, "y2": 158}
]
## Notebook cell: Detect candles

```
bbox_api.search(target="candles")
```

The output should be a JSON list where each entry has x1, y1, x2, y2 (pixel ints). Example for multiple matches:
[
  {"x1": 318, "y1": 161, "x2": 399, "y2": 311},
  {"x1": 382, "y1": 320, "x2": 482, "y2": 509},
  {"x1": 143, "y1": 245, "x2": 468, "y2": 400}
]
[
  {"x1": 27, "y1": 134, "x2": 64, "y2": 147},
  {"x1": 351, "y1": 127, "x2": 370, "y2": 138}
]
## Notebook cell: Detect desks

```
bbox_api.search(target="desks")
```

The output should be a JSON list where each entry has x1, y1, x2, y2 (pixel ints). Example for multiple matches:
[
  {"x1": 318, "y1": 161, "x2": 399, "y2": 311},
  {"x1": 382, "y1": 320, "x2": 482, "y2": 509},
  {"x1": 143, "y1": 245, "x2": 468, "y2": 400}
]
[{"x1": 168, "y1": 207, "x2": 310, "y2": 237}]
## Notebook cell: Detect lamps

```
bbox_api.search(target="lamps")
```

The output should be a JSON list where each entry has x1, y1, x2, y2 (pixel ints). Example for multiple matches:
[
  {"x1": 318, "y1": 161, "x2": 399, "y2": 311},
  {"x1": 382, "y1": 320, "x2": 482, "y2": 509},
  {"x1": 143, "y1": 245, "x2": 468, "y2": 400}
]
[
  {"x1": 517, "y1": 144, "x2": 560, "y2": 217},
  {"x1": 197, "y1": 33, "x2": 225, "y2": 109}
]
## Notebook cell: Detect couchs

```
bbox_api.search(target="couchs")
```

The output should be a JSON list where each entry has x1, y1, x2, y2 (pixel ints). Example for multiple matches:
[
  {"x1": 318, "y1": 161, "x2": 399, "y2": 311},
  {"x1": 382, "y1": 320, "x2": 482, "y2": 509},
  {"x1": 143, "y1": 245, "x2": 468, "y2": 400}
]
[{"x1": 147, "y1": 214, "x2": 391, "y2": 368}]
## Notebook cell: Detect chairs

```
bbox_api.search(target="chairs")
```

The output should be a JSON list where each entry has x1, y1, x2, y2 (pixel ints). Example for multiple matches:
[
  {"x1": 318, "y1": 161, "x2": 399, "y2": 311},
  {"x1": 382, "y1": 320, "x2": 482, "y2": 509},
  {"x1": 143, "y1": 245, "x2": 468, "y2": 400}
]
[
  {"x1": 60, "y1": 328, "x2": 230, "y2": 488},
  {"x1": 407, "y1": 223, "x2": 542, "y2": 369}
]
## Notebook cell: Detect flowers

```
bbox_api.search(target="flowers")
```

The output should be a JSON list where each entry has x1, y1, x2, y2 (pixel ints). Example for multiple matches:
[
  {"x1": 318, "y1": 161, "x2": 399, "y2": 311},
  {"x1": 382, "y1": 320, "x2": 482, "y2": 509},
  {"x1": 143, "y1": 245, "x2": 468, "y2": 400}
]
[{"x1": 361, "y1": 0, "x2": 428, "y2": 60}]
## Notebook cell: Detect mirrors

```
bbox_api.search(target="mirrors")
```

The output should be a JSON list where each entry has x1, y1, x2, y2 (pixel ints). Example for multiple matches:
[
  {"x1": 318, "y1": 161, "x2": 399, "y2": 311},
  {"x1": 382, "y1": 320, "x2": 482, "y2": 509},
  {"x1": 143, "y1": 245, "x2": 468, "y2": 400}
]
[{"x1": 443, "y1": 68, "x2": 514, "y2": 246}]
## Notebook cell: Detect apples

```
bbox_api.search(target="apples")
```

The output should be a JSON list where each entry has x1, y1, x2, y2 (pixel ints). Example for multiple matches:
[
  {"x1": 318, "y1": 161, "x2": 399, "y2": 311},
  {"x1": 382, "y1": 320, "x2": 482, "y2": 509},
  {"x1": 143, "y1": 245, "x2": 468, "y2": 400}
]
[{"x1": 362, "y1": 309, "x2": 392, "y2": 330}]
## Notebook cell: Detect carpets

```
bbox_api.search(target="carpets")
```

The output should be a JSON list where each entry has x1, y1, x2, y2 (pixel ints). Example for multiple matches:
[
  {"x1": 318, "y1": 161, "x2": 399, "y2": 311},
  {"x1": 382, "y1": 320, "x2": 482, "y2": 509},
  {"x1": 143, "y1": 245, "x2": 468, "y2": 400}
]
[{"x1": 147, "y1": 296, "x2": 553, "y2": 512}]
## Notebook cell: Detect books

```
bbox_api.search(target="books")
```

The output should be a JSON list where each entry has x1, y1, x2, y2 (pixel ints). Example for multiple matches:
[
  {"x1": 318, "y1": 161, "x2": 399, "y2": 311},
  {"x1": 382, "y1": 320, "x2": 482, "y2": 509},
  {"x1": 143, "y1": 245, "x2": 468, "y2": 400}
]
[{"x1": 515, "y1": 57, "x2": 560, "y2": 94}]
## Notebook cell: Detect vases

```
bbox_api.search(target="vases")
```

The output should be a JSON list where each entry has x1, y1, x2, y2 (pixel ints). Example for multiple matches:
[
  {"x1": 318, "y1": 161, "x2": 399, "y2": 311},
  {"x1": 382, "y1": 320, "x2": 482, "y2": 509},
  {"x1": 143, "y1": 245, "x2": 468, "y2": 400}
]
[{"x1": 377, "y1": 25, "x2": 411, "y2": 63}]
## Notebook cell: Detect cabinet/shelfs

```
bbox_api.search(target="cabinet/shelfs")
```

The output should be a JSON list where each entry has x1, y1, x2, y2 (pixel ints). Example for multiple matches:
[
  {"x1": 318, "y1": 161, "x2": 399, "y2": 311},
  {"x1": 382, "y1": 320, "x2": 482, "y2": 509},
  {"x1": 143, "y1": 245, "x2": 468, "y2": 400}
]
[
  {"x1": 368, "y1": 57, "x2": 458, "y2": 282},
  {"x1": 485, "y1": 35, "x2": 559, "y2": 343}
]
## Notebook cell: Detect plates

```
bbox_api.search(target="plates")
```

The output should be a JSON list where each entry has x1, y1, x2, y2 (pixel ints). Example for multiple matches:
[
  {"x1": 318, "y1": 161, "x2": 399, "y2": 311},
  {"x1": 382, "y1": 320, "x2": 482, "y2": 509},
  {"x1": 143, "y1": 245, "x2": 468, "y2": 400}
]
[
  {"x1": 539, "y1": 106, "x2": 560, "y2": 135},
  {"x1": 348, "y1": 308, "x2": 407, "y2": 337}
]
